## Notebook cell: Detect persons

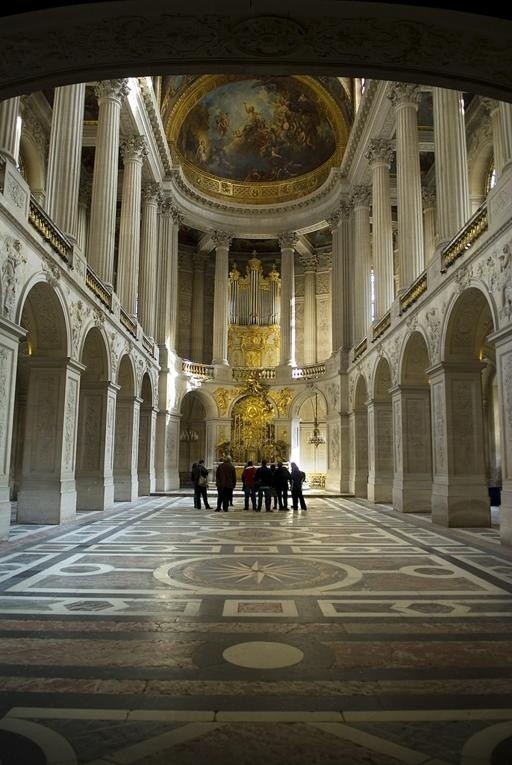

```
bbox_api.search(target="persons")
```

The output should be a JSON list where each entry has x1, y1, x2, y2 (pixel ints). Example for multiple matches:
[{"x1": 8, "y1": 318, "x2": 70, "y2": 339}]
[
  {"x1": 191, "y1": 455, "x2": 308, "y2": 512},
  {"x1": 184, "y1": 92, "x2": 334, "y2": 183}
]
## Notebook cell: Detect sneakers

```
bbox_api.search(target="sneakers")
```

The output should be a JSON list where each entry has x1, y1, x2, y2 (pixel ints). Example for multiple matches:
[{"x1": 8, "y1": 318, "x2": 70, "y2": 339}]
[
  {"x1": 194, "y1": 506, "x2": 213, "y2": 509},
  {"x1": 272, "y1": 507, "x2": 289, "y2": 511},
  {"x1": 215, "y1": 507, "x2": 228, "y2": 512},
  {"x1": 243, "y1": 508, "x2": 274, "y2": 512},
  {"x1": 292, "y1": 506, "x2": 306, "y2": 510}
]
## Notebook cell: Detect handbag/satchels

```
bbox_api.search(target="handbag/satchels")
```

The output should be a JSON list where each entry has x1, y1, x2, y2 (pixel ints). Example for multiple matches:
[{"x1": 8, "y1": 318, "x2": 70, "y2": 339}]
[{"x1": 198, "y1": 476, "x2": 207, "y2": 488}]
